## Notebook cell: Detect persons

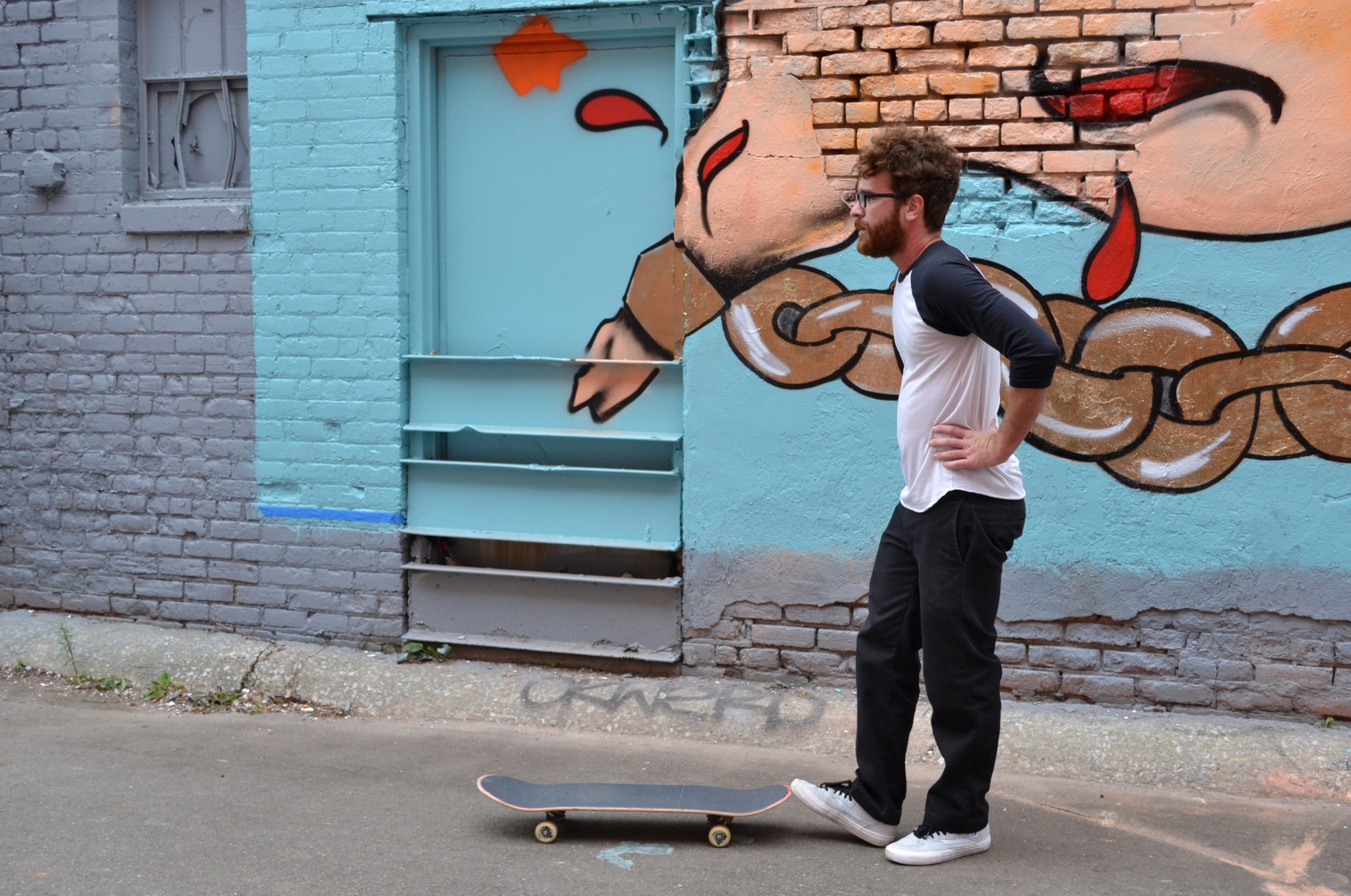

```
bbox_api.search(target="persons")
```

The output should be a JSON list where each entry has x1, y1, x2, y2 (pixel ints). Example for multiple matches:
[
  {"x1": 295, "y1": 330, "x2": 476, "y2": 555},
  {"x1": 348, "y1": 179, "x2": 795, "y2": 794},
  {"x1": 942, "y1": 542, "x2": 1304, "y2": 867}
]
[{"x1": 791, "y1": 126, "x2": 1064, "y2": 865}]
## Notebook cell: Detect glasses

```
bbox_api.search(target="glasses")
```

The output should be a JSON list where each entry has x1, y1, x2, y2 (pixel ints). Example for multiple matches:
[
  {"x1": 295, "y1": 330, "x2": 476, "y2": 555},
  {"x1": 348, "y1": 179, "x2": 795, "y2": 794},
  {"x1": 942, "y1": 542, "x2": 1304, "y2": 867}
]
[{"x1": 854, "y1": 191, "x2": 928, "y2": 210}]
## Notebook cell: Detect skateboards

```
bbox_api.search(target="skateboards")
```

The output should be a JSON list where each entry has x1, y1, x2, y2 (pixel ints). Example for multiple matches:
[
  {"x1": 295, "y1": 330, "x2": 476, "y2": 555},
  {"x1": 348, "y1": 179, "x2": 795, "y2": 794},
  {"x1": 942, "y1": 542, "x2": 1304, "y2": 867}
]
[{"x1": 478, "y1": 774, "x2": 791, "y2": 848}]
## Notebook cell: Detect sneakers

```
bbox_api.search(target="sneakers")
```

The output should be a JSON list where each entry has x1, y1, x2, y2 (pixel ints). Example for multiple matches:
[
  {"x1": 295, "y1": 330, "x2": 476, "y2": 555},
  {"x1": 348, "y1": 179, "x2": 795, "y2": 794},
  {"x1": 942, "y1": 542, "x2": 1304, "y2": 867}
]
[
  {"x1": 885, "y1": 823, "x2": 990, "y2": 866},
  {"x1": 790, "y1": 779, "x2": 898, "y2": 847}
]
